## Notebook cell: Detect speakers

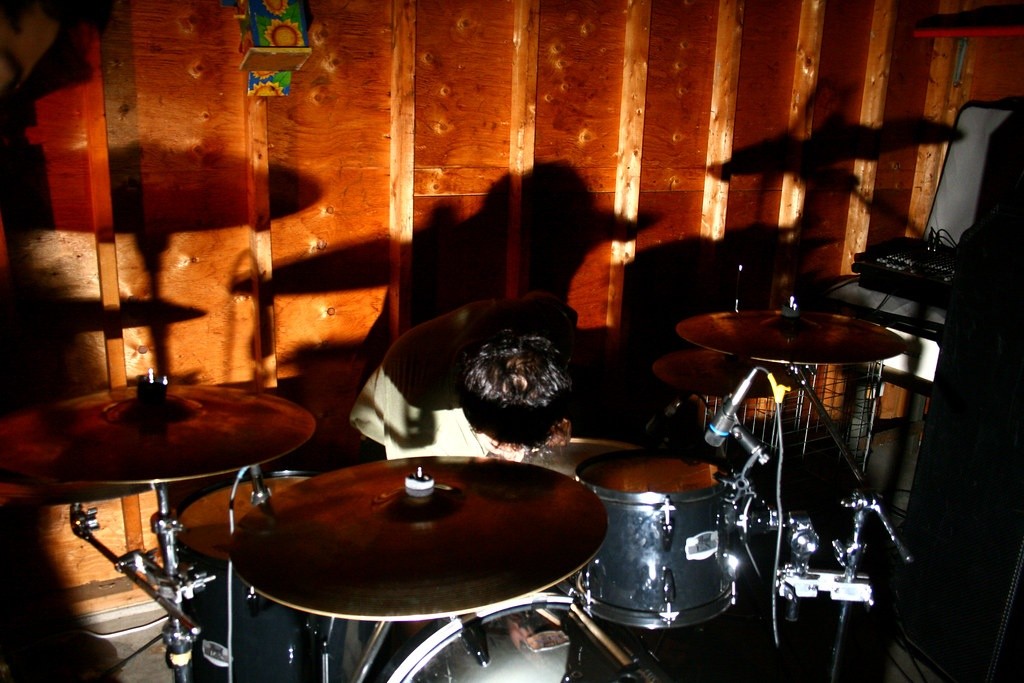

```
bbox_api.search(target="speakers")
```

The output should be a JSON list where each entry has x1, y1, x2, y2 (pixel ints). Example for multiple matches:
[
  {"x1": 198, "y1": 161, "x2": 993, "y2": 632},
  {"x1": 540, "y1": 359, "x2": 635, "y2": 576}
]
[{"x1": 890, "y1": 205, "x2": 1024, "y2": 683}]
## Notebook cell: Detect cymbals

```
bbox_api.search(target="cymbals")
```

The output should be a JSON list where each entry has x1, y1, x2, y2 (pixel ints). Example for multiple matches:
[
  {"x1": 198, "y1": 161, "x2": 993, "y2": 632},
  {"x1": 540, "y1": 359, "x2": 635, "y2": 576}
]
[
  {"x1": 652, "y1": 347, "x2": 805, "y2": 399},
  {"x1": 675, "y1": 308, "x2": 907, "y2": 365},
  {"x1": 228, "y1": 455, "x2": 610, "y2": 623},
  {"x1": 0, "y1": 382, "x2": 316, "y2": 486}
]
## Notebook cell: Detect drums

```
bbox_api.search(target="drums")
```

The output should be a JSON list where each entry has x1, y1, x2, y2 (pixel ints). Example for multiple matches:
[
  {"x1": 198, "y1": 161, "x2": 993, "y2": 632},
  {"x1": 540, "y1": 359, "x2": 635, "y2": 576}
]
[
  {"x1": 572, "y1": 448, "x2": 741, "y2": 630},
  {"x1": 369, "y1": 592, "x2": 705, "y2": 683},
  {"x1": 172, "y1": 469, "x2": 367, "y2": 683}
]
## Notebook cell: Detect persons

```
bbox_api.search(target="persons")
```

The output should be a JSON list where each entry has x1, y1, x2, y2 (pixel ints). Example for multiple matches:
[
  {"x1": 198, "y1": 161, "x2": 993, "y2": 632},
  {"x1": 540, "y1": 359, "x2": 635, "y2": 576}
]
[{"x1": 346, "y1": 288, "x2": 578, "y2": 470}]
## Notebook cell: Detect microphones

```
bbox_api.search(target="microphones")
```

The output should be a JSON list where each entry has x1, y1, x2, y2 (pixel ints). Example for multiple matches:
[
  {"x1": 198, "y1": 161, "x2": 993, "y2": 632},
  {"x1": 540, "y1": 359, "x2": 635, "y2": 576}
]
[
  {"x1": 249, "y1": 463, "x2": 273, "y2": 506},
  {"x1": 705, "y1": 367, "x2": 758, "y2": 448}
]
